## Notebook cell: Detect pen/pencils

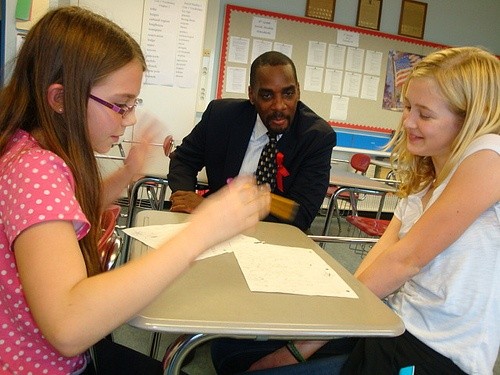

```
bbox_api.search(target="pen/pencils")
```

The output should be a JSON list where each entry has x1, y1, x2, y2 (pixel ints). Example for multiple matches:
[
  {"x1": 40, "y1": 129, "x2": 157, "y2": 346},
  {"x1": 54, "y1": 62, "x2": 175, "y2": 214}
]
[{"x1": 227, "y1": 177, "x2": 301, "y2": 222}]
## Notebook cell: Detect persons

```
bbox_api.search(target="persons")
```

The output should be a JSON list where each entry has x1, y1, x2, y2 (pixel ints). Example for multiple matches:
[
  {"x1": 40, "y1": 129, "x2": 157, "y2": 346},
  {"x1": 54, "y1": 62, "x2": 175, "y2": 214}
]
[
  {"x1": 210, "y1": 46, "x2": 500, "y2": 375},
  {"x1": 0, "y1": 6, "x2": 272, "y2": 375},
  {"x1": 166, "y1": 53, "x2": 336, "y2": 232}
]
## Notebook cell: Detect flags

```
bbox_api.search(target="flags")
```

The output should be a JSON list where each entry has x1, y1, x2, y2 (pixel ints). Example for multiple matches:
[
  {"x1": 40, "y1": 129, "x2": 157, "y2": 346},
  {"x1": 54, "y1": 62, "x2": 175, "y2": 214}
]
[{"x1": 393, "y1": 54, "x2": 421, "y2": 87}]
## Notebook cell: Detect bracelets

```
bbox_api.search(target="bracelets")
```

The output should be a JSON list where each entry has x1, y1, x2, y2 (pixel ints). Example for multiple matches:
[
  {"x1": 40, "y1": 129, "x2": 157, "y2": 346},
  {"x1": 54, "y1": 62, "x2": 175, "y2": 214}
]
[{"x1": 287, "y1": 340, "x2": 305, "y2": 363}]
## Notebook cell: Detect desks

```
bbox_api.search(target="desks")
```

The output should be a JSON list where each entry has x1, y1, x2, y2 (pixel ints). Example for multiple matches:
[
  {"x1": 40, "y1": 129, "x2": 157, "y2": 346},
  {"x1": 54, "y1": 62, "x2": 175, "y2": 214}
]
[
  {"x1": 320, "y1": 171, "x2": 395, "y2": 250},
  {"x1": 121, "y1": 211, "x2": 406, "y2": 374}
]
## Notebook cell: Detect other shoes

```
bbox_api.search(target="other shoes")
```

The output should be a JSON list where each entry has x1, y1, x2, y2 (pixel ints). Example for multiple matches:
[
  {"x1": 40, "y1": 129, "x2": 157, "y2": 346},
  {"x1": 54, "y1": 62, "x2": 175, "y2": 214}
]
[{"x1": 162, "y1": 333, "x2": 196, "y2": 369}]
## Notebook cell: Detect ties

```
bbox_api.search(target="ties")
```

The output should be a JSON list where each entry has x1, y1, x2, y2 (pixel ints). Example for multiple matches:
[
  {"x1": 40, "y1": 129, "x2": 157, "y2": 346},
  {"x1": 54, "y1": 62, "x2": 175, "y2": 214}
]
[{"x1": 254, "y1": 132, "x2": 279, "y2": 194}]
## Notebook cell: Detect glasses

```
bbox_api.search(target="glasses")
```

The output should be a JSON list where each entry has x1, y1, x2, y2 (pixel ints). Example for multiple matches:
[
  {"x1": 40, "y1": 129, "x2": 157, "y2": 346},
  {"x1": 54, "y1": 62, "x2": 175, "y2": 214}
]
[{"x1": 53, "y1": 81, "x2": 144, "y2": 119}]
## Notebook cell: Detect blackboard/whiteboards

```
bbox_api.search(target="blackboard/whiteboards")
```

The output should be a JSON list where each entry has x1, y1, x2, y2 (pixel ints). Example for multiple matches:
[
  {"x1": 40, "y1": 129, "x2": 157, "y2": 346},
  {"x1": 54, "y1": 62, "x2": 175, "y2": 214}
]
[{"x1": 70, "y1": 0, "x2": 208, "y2": 201}]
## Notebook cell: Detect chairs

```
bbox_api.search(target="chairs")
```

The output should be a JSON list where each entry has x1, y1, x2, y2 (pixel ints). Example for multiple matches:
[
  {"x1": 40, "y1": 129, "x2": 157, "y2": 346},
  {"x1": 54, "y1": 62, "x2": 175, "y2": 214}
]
[
  {"x1": 322, "y1": 153, "x2": 396, "y2": 259},
  {"x1": 115, "y1": 134, "x2": 178, "y2": 212}
]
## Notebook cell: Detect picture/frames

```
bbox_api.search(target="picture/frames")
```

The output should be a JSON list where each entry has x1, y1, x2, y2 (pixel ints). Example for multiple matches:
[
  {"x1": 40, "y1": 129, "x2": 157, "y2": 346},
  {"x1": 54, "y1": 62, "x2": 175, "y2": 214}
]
[
  {"x1": 306, "y1": 0, "x2": 336, "y2": 21},
  {"x1": 356, "y1": 0, "x2": 383, "y2": 30},
  {"x1": 398, "y1": 0, "x2": 428, "y2": 39}
]
